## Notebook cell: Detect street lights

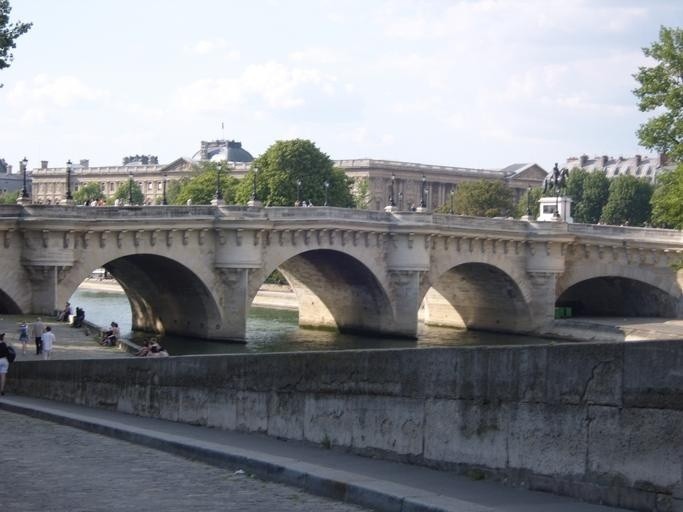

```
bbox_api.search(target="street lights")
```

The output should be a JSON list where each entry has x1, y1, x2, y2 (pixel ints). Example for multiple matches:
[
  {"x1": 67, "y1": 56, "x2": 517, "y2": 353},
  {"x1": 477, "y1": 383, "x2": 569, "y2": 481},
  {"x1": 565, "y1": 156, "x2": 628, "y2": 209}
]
[
  {"x1": 64, "y1": 160, "x2": 73, "y2": 200},
  {"x1": 323, "y1": 178, "x2": 329, "y2": 206},
  {"x1": 18, "y1": 157, "x2": 30, "y2": 198},
  {"x1": 216, "y1": 162, "x2": 222, "y2": 200},
  {"x1": 161, "y1": 175, "x2": 167, "y2": 205},
  {"x1": 553, "y1": 189, "x2": 560, "y2": 217},
  {"x1": 526, "y1": 184, "x2": 532, "y2": 215},
  {"x1": 449, "y1": 188, "x2": 454, "y2": 214},
  {"x1": 391, "y1": 174, "x2": 395, "y2": 206},
  {"x1": 295, "y1": 179, "x2": 301, "y2": 207},
  {"x1": 421, "y1": 175, "x2": 426, "y2": 207},
  {"x1": 252, "y1": 165, "x2": 259, "y2": 201},
  {"x1": 128, "y1": 171, "x2": 134, "y2": 205}
]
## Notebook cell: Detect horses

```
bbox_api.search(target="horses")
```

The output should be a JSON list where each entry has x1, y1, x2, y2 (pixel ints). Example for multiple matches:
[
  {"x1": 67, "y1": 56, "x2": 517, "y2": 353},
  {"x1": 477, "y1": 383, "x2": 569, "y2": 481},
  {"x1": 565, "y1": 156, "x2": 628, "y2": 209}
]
[{"x1": 541, "y1": 168, "x2": 570, "y2": 197}]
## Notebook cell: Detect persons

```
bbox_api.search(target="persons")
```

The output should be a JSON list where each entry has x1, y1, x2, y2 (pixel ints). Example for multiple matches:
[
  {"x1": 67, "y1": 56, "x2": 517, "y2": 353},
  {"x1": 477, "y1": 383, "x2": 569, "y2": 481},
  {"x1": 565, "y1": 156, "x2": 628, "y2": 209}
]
[
  {"x1": 41, "y1": 326, "x2": 57, "y2": 360},
  {"x1": 19, "y1": 320, "x2": 31, "y2": 354},
  {"x1": 295, "y1": 199, "x2": 314, "y2": 208},
  {"x1": 104, "y1": 324, "x2": 120, "y2": 343},
  {"x1": 552, "y1": 163, "x2": 560, "y2": 190},
  {"x1": 100, "y1": 322, "x2": 114, "y2": 345},
  {"x1": 0, "y1": 333, "x2": 13, "y2": 396},
  {"x1": 134, "y1": 337, "x2": 162, "y2": 357},
  {"x1": 84, "y1": 197, "x2": 125, "y2": 207},
  {"x1": 56, "y1": 302, "x2": 85, "y2": 329},
  {"x1": 32, "y1": 317, "x2": 45, "y2": 354},
  {"x1": 161, "y1": 199, "x2": 167, "y2": 205}
]
